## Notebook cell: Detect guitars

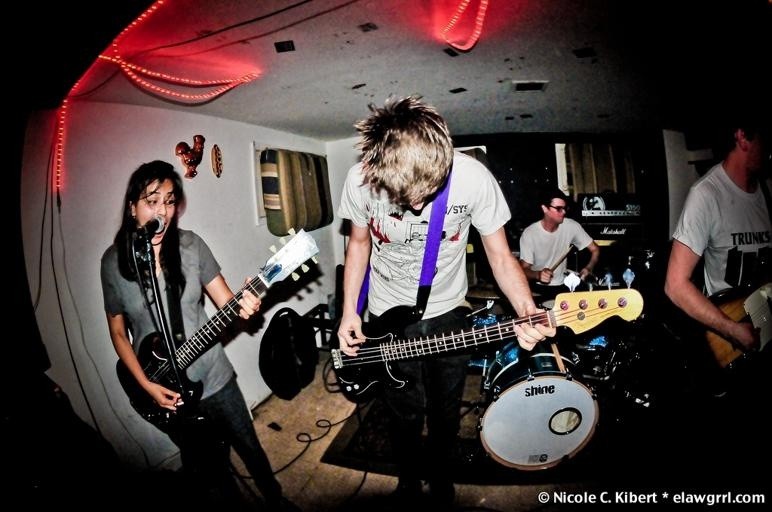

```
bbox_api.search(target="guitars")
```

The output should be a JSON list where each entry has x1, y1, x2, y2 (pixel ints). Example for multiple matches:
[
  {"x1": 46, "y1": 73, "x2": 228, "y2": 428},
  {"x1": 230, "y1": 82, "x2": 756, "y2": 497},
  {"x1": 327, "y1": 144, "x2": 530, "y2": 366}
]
[
  {"x1": 116, "y1": 229, "x2": 318, "y2": 426},
  {"x1": 329, "y1": 268, "x2": 646, "y2": 404},
  {"x1": 693, "y1": 285, "x2": 772, "y2": 397}
]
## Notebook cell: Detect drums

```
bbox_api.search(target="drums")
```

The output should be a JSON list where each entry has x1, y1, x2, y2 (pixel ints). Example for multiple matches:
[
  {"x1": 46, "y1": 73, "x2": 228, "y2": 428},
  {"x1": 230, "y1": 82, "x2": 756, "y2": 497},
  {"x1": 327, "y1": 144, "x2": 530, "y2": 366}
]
[
  {"x1": 570, "y1": 340, "x2": 621, "y2": 382},
  {"x1": 479, "y1": 341, "x2": 597, "y2": 471}
]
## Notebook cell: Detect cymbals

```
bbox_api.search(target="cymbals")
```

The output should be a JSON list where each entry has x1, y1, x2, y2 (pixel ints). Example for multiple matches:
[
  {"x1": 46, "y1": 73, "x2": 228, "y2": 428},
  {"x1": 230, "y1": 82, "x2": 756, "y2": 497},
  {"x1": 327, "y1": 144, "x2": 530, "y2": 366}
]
[{"x1": 464, "y1": 283, "x2": 542, "y2": 300}]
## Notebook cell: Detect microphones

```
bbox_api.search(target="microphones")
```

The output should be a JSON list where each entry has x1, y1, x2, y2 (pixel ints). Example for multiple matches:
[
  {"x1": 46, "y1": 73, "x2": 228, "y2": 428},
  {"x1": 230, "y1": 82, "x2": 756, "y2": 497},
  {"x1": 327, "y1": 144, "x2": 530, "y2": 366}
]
[{"x1": 129, "y1": 215, "x2": 165, "y2": 241}]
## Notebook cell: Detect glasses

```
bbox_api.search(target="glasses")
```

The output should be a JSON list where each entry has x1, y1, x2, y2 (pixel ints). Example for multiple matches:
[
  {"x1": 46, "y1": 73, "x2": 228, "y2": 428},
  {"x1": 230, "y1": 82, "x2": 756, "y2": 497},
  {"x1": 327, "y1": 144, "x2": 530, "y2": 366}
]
[{"x1": 549, "y1": 205, "x2": 569, "y2": 212}]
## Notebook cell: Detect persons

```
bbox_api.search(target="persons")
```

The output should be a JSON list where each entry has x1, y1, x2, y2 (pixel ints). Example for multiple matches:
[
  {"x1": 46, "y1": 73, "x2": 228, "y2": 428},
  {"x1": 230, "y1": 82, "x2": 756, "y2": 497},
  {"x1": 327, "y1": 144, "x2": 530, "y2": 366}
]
[
  {"x1": 100, "y1": 161, "x2": 300, "y2": 510},
  {"x1": 337, "y1": 98, "x2": 558, "y2": 512},
  {"x1": 662, "y1": 113, "x2": 770, "y2": 512},
  {"x1": 520, "y1": 194, "x2": 601, "y2": 302}
]
